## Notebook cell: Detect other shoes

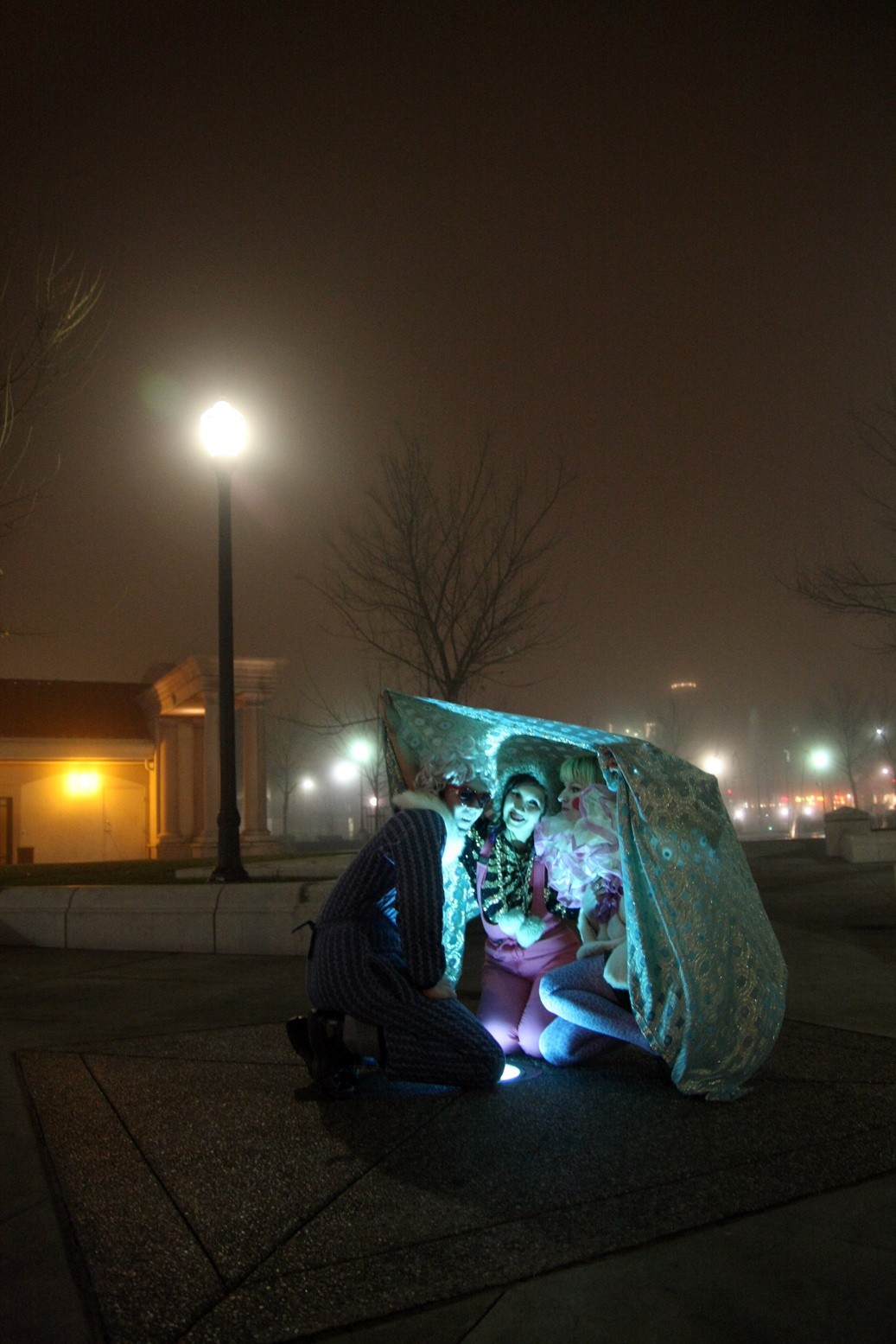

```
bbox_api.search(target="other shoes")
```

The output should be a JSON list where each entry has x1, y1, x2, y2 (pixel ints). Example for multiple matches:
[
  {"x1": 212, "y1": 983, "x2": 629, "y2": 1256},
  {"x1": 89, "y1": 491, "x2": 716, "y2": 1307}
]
[
  {"x1": 308, "y1": 1002, "x2": 365, "y2": 1101},
  {"x1": 286, "y1": 1015, "x2": 326, "y2": 1078}
]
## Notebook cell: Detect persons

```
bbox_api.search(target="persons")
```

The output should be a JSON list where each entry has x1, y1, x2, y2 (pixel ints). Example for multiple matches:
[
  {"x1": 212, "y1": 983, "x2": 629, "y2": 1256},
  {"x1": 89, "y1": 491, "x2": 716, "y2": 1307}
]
[{"x1": 283, "y1": 724, "x2": 663, "y2": 1087}]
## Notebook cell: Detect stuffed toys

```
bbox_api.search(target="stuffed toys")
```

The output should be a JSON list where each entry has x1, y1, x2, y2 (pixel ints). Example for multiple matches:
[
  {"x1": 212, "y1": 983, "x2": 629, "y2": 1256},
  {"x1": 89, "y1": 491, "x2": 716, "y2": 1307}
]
[{"x1": 544, "y1": 785, "x2": 630, "y2": 992}]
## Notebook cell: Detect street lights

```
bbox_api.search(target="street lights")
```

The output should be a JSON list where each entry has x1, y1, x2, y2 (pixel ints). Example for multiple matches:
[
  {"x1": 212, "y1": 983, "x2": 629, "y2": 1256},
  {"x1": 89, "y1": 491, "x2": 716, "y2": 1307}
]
[{"x1": 200, "y1": 402, "x2": 255, "y2": 885}]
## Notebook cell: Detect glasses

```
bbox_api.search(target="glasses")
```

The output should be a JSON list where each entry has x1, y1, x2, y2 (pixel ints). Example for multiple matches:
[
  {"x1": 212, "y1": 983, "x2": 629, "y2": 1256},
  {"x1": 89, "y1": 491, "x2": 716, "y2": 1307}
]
[{"x1": 455, "y1": 787, "x2": 491, "y2": 808}]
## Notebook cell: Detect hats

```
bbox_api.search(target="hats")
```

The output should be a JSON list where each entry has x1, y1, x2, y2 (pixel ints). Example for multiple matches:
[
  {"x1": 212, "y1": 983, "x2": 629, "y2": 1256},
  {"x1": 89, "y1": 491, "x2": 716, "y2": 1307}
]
[{"x1": 492, "y1": 756, "x2": 563, "y2": 948}]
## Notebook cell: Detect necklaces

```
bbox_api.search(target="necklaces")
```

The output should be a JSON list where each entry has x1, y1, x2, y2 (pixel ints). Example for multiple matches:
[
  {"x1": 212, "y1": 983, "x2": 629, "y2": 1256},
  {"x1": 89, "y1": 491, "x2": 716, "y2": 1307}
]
[{"x1": 493, "y1": 831, "x2": 533, "y2": 922}]
